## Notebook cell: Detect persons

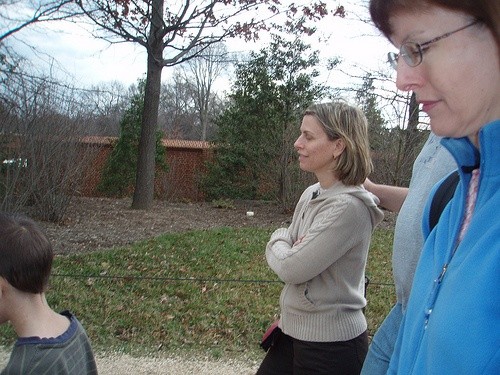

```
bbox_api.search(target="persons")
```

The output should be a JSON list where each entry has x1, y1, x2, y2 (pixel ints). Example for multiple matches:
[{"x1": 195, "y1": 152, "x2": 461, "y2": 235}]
[
  {"x1": 360, "y1": 175, "x2": 411, "y2": 214},
  {"x1": 0, "y1": 214, "x2": 99, "y2": 375},
  {"x1": 255, "y1": 103, "x2": 384, "y2": 375},
  {"x1": 357, "y1": 0, "x2": 500, "y2": 375}
]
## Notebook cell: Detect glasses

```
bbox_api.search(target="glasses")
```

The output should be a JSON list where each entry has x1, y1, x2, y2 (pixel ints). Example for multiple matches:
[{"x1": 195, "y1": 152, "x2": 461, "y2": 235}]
[{"x1": 385, "y1": 20, "x2": 489, "y2": 72}]
